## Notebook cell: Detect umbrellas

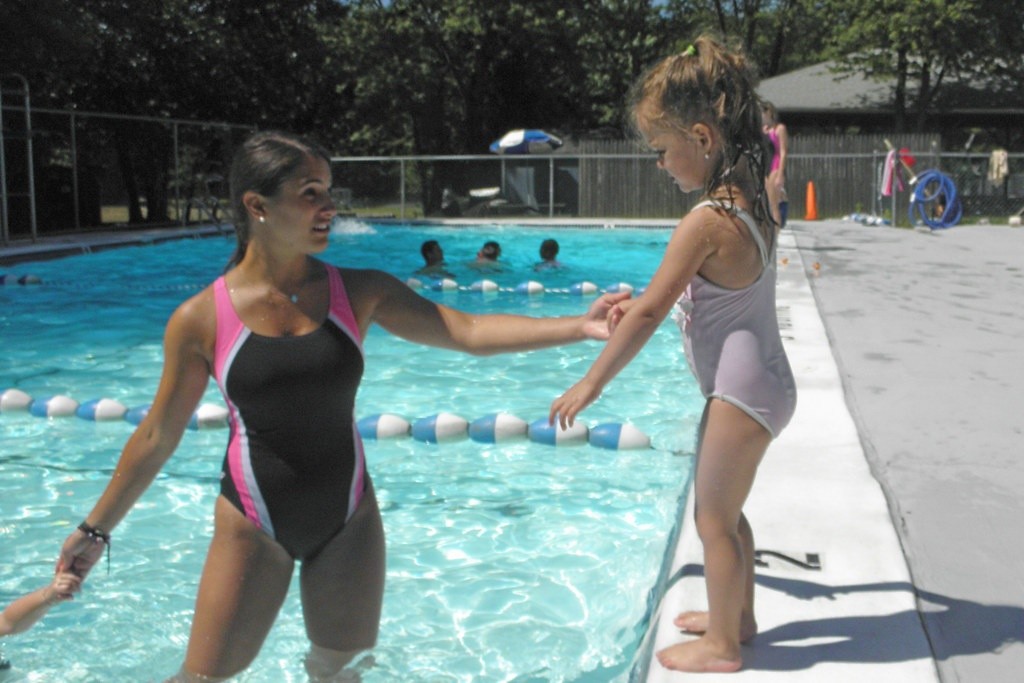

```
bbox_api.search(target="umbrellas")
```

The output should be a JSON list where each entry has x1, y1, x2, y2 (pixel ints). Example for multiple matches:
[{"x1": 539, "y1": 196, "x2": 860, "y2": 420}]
[{"x1": 489, "y1": 129, "x2": 563, "y2": 202}]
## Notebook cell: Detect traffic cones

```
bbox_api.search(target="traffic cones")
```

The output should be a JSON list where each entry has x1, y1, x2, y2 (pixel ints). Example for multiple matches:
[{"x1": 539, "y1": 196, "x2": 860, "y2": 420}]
[{"x1": 803, "y1": 181, "x2": 820, "y2": 221}]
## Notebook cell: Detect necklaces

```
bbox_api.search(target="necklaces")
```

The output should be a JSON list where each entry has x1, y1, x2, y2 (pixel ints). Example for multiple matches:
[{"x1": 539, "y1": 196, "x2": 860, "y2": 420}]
[{"x1": 268, "y1": 272, "x2": 307, "y2": 303}]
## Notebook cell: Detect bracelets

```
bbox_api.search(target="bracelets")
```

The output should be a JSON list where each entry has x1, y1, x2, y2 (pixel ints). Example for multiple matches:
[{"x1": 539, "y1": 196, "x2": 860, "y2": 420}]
[{"x1": 78, "y1": 522, "x2": 113, "y2": 570}]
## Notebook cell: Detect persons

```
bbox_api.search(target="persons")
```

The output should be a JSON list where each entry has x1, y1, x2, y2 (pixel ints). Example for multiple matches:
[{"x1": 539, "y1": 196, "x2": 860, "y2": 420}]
[
  {"x1": 760, "y1": 99, "x2": 787, "y2": 242},
  {"x1": 0, "y1": 573, "x2": 81, "y2": 635},
  {"x1": 548, "y1": 33, "x2": 798, "y2": 672},
  {"x1": 415, "y1": 235, "x2": 570, "y2": 279},
  {"x1": 54, "y1": 130, "x2": 632, "y2": 683}
]
